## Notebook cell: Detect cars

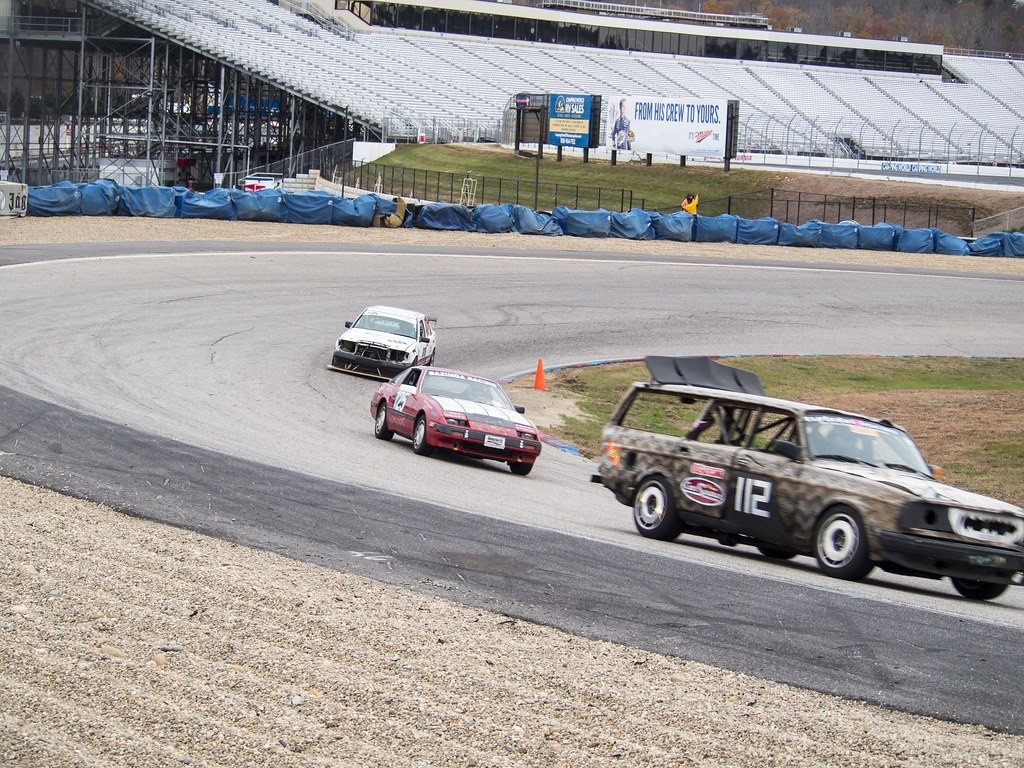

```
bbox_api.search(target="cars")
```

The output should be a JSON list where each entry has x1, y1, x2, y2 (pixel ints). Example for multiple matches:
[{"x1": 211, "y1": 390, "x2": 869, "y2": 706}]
[
  {"x1": 595, "y1": 380, "x2": 1024, "y2": 600},
  {"x1": 329, "y1": 305, "x2": 438, "y2": 385},
  {"x1": 371, "y1": 365, "x2": 542, "y2": 476}
]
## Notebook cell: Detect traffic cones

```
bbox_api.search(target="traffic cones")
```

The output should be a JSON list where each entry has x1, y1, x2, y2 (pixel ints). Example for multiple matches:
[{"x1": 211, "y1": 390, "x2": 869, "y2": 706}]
[{"x1": 534, "y1": 358, "x2": 548, "y2": 391}]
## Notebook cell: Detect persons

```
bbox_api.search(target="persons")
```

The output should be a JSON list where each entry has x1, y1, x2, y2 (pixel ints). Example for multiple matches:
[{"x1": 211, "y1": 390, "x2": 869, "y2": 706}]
[
  {"x1": 394, "y1": 321, "x2": 414, "y2": 336},
  {"x1": 261, "y1": 140, "x2": 291, "y2": 163},
  {"x1": 611, "y1": 97, "x2": 635, "y2": 150},
  {"x1": 465, "y1": 383, "x2": 491, "y2": 404},
  {"x1": 824, "y1": 424, "x2": 863, "y2": 461},
  {"x1": 680, "y1": 193, "x2": 699, "y2": 216}
]
